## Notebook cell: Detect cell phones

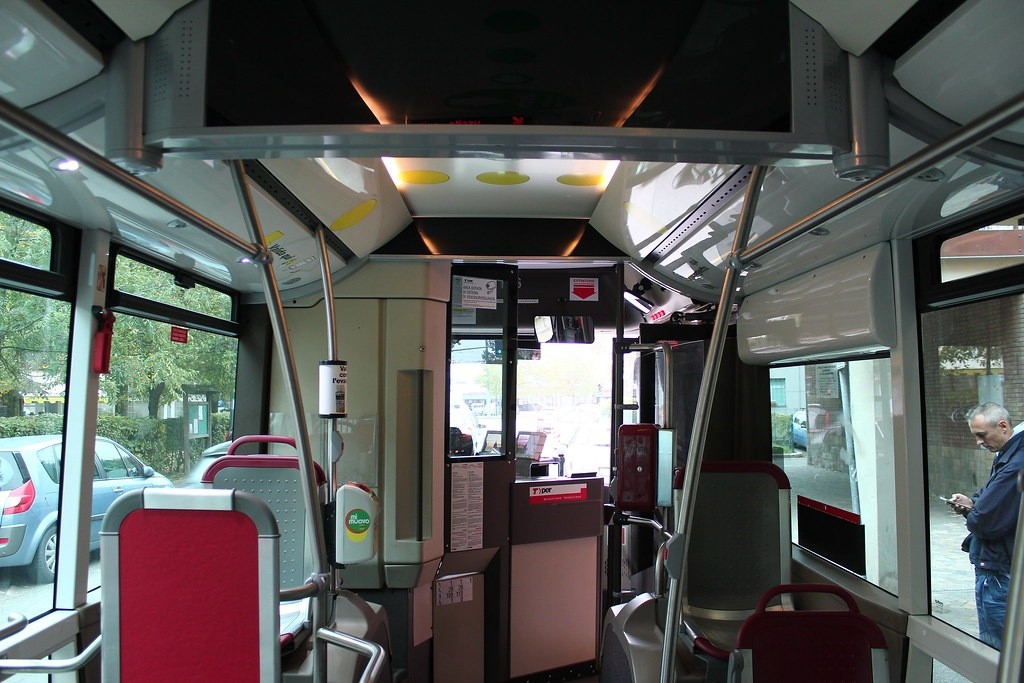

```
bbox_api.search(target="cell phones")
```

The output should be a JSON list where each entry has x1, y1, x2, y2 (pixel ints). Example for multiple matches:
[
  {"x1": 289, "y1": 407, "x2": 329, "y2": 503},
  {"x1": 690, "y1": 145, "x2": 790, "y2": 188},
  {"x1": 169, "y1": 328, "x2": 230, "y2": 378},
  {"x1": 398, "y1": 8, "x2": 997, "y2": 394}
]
[{"x1": 939, "y1": 495, "x2": 971, "y2": 510}]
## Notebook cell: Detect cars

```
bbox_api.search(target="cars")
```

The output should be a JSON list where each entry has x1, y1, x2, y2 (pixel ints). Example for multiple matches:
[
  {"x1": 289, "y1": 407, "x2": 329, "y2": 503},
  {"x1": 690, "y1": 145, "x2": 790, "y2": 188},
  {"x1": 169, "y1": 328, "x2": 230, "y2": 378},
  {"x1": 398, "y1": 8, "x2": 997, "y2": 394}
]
[{"x1": 788, "y1": 409, "x2": 807, "y2": 451}]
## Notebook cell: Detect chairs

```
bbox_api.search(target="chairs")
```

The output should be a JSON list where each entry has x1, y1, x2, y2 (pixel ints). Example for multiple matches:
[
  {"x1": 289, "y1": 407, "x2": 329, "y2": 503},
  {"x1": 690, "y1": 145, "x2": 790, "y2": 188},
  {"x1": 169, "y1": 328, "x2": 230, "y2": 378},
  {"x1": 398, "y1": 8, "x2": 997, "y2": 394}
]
[
  {"x1": 192, "y1": 432, "x2": 326, "y2": 660},
  {"x1": 102, "y1": 485, "x2": 281, "y2": 683},
  {"x1": 672, "y1": 462, "x2": 795, "y2": 661},
  {"x1": 728, "y1": 585, "x2": 890, "y2": 683}
]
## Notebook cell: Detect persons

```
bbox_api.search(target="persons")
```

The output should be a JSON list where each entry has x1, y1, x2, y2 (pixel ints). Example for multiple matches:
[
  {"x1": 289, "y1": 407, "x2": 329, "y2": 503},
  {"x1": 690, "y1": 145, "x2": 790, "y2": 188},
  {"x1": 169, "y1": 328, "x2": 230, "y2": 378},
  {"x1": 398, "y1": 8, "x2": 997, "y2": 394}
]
[{"x1": 945, "y1": 401, "x2": 1024, "y2": 651}]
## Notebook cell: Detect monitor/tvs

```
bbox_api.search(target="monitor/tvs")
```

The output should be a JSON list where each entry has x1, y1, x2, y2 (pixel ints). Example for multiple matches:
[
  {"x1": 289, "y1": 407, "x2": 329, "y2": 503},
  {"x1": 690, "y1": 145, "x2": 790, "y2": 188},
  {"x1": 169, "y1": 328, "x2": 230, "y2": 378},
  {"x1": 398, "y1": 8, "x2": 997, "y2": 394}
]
[{"x1": 199, "y1": 4, "x2": 801, "y2": 138}]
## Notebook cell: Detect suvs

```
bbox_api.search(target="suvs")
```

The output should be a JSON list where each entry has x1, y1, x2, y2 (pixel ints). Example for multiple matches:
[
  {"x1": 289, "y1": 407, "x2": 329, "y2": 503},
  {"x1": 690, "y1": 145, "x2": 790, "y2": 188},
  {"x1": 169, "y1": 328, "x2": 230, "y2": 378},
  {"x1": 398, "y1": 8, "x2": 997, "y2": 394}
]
[{"x1": 0, "y1": 435, "x2": 176, "y2": 587}]
